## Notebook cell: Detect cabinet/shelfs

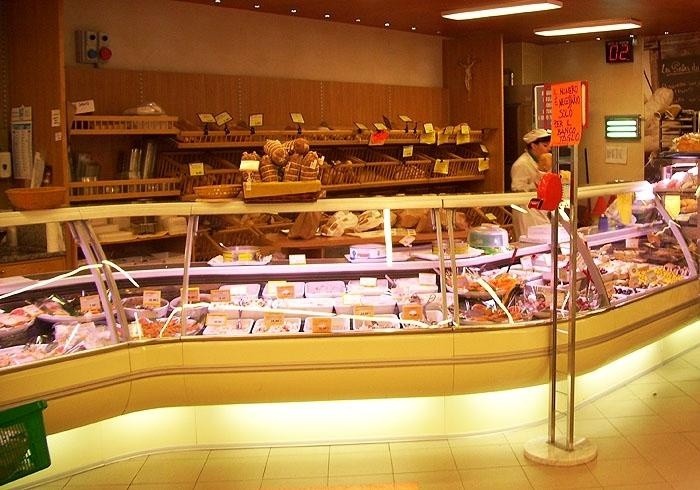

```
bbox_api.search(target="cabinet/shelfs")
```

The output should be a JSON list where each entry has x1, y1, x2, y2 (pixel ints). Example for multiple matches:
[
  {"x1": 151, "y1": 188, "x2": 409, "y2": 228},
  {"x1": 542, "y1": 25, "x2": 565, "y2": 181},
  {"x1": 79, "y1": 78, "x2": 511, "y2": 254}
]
[{"x1": 0, "y1": 114, "x2": 699, "y2": 445}]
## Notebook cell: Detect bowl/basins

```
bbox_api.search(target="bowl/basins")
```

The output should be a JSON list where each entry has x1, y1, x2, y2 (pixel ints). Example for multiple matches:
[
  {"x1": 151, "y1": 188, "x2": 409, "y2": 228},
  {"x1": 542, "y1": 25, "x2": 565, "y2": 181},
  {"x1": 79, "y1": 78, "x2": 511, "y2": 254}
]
[{"x1": 119, "y1": 293, "x2": 211, "y2": 319}]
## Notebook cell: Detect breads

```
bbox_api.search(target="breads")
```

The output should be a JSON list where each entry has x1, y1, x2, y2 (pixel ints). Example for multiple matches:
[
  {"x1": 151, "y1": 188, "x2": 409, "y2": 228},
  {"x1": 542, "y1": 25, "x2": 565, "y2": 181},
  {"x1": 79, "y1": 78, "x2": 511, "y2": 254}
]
[{"x1": 241, "y1": 137, "x2": 320, "y2": 181}]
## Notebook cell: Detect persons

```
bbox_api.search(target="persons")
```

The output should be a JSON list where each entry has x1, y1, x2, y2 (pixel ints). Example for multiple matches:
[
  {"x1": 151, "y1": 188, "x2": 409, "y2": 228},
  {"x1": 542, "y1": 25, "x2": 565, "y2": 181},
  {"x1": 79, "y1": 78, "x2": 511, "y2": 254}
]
[{"x1": 510, "y1": 127, "x2": 551, "y2": 241}]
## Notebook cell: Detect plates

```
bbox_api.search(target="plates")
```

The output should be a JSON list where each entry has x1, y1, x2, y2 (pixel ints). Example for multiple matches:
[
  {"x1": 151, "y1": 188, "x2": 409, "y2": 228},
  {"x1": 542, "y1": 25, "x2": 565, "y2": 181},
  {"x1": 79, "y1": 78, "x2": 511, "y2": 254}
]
[
  {"x1": 202, "y1": 280, "x2": 449, "y2": 337},
  {"x1": 35, "y1": 306, "x2": 119, "y2": 324}
]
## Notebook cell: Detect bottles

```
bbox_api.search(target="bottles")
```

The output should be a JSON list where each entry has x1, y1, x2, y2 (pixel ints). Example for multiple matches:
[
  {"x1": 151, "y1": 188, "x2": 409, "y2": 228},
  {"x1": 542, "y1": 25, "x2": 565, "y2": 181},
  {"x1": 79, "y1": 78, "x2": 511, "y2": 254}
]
[{"x1": 598, "y1": 213, "x2": 608, "y2": 233}]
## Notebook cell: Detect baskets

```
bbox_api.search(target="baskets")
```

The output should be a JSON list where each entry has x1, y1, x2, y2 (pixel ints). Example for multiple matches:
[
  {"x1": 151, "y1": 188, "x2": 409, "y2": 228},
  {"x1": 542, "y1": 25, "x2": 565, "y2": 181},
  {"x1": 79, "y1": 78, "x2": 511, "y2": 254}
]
[
  {"x1": 675, "y1": 138, "x2": 700, "y2": 155},
  {"x1": 454, "y1": 206, "x2": 514, "y2": 242},
  {"x1": 5, "y1": 187, "x2": 66, "y2": 211},
  {"x1": 154, "y1": 151, "x2": 242, "y2": 194},
  {"x1": 0, "y1": 299, "x2": 41, "y2": 350},
  {"x1": 311, "y1": 145, "x2": 485, "y2": 185},
  {"x1": 195, "y1": 212, "x2": 328, "y2": 261},
  {"x1": 193, "y1": 183, "x2": 242, "y2": 199},
  {"x1": 243, "y1": 180, "x2": 321, "y2": 204},
  {"x1": 0, "y1": 400, "x2": 51, "y2": 486}
]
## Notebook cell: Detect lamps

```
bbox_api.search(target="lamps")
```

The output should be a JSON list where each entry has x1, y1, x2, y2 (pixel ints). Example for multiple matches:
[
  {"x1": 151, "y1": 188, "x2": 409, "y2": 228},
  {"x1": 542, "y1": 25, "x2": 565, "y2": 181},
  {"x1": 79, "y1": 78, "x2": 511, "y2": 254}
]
[{"x1": 440, "y1": 0, "x2": 642, "y2": 38}]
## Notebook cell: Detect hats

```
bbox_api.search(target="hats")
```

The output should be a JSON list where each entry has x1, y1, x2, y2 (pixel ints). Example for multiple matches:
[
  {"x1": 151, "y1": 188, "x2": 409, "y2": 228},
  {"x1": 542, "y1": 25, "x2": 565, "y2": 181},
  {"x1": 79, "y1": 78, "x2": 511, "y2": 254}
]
[{"x1": 522, "y1": 129, "x2": 551, "y2": 145}]
